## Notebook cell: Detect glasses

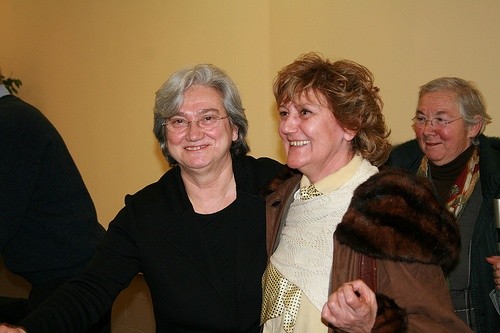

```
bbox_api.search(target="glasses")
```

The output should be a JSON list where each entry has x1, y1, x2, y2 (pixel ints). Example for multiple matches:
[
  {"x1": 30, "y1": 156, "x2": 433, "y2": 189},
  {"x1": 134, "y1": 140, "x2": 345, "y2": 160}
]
[
  {"x1": 161, "y1": 114, "x2": 229, "y2": 133},
  {"x1": 411, "y1": 115, "x2": 461, "y2": 131}
]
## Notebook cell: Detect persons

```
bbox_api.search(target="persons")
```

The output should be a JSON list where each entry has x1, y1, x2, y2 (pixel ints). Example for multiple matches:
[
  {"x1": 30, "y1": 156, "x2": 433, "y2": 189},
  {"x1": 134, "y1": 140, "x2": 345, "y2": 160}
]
[
  {"x1": 258, "y1": 51, "x2": 474, "y2": 333},
  {"x1": 0, "y1": 65, "x2": 304, "y2": 333},
  {"x1": 382, "y1": 78, "x2": 500, "y2": 333}
]
